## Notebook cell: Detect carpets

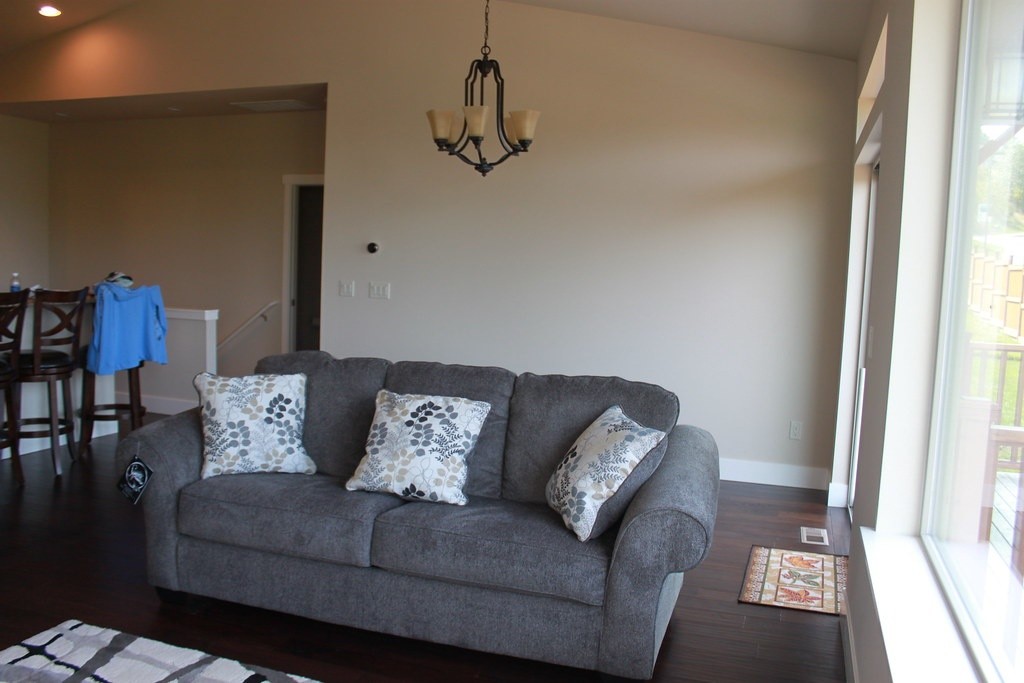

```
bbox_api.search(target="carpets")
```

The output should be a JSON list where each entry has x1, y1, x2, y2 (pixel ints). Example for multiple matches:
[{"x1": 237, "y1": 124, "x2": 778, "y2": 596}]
[
  {"x1": 736, "y1": 545, "x2": 851, "y2": 617},
  {"x1": 0, "y1": 620, "x2": 320, "y2": 683}
]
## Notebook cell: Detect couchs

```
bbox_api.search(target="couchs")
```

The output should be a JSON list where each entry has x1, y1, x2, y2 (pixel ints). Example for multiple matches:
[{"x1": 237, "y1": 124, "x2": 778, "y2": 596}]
[{"x1": 113, "y1": 354, "x2": 721, "y2": 681}]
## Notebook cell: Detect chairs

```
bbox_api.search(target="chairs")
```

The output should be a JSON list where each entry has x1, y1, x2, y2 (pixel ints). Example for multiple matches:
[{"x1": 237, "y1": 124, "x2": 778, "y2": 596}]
[
  {"x1": 76, "y1": 282, "x2": 170, "y2": 461},
  {"x1": 0, "y1": 288, "x2": 32, "y2": 459},
  {"x1": 0, "y1": 287, "x2": 91, "y2": 476}
]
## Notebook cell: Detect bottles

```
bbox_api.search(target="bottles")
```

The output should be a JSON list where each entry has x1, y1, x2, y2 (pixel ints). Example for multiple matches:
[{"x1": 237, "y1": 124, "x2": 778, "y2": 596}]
[{"x1": 10, "y1": 272, "x2": 21, "y2": 292}]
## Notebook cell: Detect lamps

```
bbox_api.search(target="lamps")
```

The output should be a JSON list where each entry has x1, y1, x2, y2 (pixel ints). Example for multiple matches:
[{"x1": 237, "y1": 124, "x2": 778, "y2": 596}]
[{"x1": 426, "y1": 0, "x2": 540, "y2": 178}]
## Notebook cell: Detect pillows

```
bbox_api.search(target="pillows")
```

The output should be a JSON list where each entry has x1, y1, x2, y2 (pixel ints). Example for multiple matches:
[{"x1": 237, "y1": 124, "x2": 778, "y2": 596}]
[
  {"x1": 545, "y1": 402, "x2": 669, "y2": 544},
  {"x1": 192, "y1": 370, "x2": 318, "y2": 480},
  {"x1": 346, "y1": 390, "x2": 493, "y2": 506}
]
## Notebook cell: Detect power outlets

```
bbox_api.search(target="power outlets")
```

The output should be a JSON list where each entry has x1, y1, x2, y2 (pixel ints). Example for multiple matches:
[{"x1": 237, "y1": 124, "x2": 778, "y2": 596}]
[{"x1": 789, "y1": 421, "x2": 803, "y2": 441}]
